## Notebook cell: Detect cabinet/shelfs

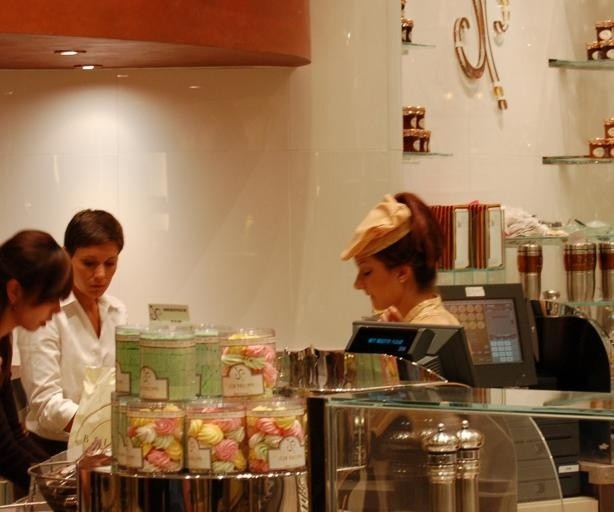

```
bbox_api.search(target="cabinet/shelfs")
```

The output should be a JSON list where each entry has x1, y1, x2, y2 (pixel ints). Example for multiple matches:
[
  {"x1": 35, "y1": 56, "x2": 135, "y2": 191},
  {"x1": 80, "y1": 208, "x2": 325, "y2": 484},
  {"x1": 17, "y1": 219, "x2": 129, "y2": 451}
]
[
  {"x1": 402, "y1": 42, "x2": 451, "y2": 155},
  {"x1": 542, "y1": 60, "x2": 614, "y2": 164}
]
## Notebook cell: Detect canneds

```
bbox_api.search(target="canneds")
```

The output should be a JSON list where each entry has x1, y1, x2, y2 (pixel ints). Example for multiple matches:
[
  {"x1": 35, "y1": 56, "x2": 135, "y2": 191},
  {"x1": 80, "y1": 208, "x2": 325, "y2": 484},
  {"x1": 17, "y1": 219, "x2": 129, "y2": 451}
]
[
  {"x1": 400, "y1": 0, "x2": 415, "y2": 42},
  {"x1": 403, "y1": 105, "x2": 431, "y2": 152},
  {"x1": 589, "y1": 116, "x2": 614, "y2": 158},
  {"x1": 586, "y1": 20, "x2": 614, "y2": 60}
]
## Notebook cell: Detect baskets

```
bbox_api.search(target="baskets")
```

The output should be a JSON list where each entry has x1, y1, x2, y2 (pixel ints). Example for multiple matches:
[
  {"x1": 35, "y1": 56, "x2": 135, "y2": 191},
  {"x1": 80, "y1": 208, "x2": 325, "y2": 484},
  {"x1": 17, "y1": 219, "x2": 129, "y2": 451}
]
[{"x1": 29, "y1": 461, "x2": 78, "y2": 511}]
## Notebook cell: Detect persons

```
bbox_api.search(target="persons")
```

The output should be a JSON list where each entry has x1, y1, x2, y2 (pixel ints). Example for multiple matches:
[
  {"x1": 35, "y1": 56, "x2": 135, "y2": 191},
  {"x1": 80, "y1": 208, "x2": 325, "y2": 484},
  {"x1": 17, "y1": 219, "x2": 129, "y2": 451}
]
[
  {"x1": 16, "y1": 210, "x2": 129, "y2": 455},
  {"x1": 2, "y1": 229, "x2": 74, "y2": 494},
  {"x1": 341, "y1": 190, "x2": 462, "y2": 326}
]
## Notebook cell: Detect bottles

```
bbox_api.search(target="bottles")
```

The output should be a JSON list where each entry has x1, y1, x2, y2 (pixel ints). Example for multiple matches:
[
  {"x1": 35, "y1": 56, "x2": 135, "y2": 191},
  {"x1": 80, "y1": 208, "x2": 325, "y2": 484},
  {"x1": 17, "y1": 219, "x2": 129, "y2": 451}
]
[
  {"x1": 513, "y1": 237, "x2": 613, "y2": 324},
  {"x1": 109, "y1": 321, "x2": 313, "y2": 482}
]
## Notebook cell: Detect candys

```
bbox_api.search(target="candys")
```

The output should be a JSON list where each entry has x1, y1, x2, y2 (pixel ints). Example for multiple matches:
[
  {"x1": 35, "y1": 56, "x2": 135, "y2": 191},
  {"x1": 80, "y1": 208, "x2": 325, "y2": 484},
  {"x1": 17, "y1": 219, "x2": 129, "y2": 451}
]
[
  {"x1": 187, "y1": 405, "x2": 248, "y2": 474},
  {"x1": 220, "y1": 332, "x2": 278, "y2": 399},
  {"x1": 126, "y1": 403, "x2": 184, "y2": 473},
  {"x1": 248, "y1": 402, "x2": 308, "y2": 473}
]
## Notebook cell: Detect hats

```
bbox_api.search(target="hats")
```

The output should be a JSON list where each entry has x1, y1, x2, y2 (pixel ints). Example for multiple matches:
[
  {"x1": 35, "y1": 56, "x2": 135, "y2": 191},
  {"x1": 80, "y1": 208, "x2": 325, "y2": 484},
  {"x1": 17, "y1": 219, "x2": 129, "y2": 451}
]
[{"x1": 340, "y1": 193, "x2": 412, "y2": 263}]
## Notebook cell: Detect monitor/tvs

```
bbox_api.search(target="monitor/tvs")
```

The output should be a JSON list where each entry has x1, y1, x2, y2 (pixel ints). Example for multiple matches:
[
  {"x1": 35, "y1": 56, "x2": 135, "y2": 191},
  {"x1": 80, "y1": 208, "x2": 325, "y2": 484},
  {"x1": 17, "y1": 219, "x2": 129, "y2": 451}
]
[
  {"x1": 437, "y1": 282, "x2": 538, "y2": 388},
  {"x1": 352, "y1": 320, "x2": 477, "y2": 389}
]
